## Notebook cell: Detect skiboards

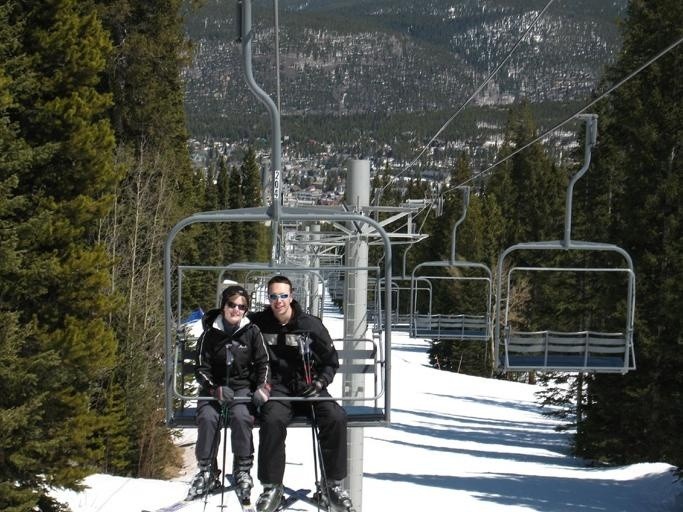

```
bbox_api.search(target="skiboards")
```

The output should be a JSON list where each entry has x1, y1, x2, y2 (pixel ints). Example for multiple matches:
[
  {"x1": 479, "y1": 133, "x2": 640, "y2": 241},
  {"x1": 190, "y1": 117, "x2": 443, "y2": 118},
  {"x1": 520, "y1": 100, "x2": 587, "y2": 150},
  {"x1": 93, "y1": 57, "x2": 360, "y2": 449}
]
[
  {"x1": 275, "y1": 481, "x2": 357, "y2": 512},
  {"x1": 141, "y1": 471, "x2": 257, "y2": 512}
]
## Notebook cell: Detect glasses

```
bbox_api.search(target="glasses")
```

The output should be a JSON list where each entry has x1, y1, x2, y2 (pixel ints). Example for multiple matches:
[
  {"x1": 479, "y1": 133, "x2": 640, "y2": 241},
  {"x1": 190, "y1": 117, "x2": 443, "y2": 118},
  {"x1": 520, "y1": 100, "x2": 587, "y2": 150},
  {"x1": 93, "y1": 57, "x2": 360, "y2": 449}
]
[
  {"x1": 268, "y1": 293, "x2": 289, "y2": 299},
  {"x1": 227, "y1": 301, "x2": 249, "y2": 310}
]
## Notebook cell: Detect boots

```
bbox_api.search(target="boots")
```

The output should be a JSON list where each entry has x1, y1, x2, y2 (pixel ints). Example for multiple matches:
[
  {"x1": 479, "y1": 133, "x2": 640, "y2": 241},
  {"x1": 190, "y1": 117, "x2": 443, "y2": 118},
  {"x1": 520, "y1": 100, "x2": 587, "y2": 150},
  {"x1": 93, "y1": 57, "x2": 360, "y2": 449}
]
[
  {"x1": 232, "y1": 455, "x2": 255, "y2": 488},
  {"x1": 192, "y1": 459, "x2": 221, "y2": 493}
]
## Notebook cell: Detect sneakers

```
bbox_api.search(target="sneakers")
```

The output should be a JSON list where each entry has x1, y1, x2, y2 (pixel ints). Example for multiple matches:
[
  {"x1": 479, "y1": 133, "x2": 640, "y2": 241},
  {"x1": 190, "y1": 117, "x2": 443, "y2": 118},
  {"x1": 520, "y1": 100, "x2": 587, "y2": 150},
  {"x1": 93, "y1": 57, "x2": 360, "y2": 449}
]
[
  {"x1": 324, "y1": 478, "x2": 352, "y2": 508},
  {"x1": 256, "y1": 481, "x2": 285, "y2": 512}
]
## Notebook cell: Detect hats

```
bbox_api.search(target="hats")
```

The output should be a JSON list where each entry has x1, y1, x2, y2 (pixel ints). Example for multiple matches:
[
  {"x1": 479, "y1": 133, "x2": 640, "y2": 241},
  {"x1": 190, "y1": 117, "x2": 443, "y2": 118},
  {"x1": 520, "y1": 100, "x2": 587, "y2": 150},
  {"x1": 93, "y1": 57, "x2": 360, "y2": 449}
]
[{"x1": 223, "y1": 286, "x2": 248, "y2": 303}]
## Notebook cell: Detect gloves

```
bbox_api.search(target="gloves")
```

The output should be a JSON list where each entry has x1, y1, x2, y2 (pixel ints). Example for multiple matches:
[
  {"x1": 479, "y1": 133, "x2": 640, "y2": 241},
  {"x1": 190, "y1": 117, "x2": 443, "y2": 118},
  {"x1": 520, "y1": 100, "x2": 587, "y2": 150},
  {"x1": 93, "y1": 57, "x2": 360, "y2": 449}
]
[
  {"x1": 253, "y1": 387, "x2": 270, "y2": 407},
  {"x1": 215, "y1": 386, "x2": 234, "y2": 401},
  {"x1": 303, "y1": 378, "x2": 323, "y2": 398}
]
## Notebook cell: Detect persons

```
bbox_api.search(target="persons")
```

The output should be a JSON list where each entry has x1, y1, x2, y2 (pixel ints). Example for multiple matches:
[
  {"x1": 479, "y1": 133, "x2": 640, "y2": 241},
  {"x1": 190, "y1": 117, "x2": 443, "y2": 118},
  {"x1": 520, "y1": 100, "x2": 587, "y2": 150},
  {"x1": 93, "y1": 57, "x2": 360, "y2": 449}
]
[
  {"x1": 180, "y1": 286, "x2": 272, "y2": 500},
  {"x1": 247, "y1": 276, "x2": 353, "y2": 512}
]
